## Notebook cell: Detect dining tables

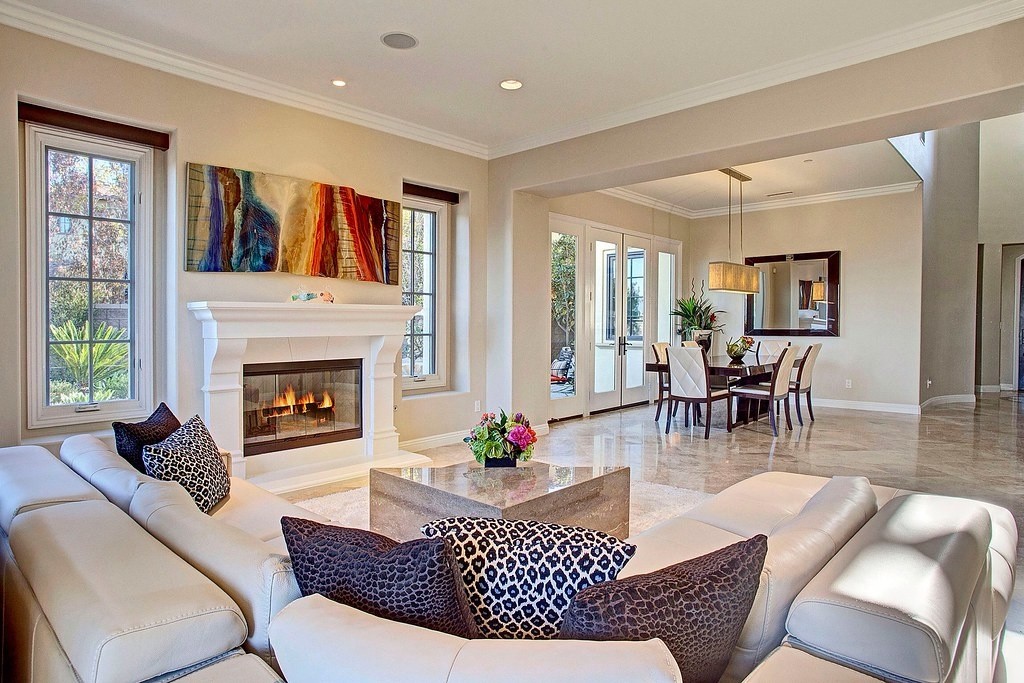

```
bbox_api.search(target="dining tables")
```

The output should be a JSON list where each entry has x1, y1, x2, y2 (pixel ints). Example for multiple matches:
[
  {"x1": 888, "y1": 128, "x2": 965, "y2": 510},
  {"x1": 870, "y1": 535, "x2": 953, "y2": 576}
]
[{"x1": 645, "y1": 353, "x2": 781, "y2": 428}]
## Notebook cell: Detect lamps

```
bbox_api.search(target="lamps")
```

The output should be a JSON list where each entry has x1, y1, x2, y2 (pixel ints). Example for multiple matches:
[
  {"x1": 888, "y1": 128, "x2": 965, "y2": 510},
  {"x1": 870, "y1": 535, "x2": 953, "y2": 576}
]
[{"x1": 708, "y1": 167, "x2": 760, "y2": 294}]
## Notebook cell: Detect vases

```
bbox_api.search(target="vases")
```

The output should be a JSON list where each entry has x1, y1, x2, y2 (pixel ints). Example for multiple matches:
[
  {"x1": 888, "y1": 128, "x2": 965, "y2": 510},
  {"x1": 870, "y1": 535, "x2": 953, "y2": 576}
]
[
  {"x1": 726, "y1": 352, "x2": 745, "y2": 364},
  {"x1": 484, "y1": 453, "x2": 515, "y2": 468},
  {"x1": 690, "y1": 330, "x2": 713, "y2": 354}
]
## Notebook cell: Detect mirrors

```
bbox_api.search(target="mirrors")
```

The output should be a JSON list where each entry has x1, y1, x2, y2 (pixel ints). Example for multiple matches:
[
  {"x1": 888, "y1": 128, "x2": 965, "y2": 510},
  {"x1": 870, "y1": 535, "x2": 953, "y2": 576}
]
[{"x1": 743, "y1": 250, "x2": 841, "y2": 337}]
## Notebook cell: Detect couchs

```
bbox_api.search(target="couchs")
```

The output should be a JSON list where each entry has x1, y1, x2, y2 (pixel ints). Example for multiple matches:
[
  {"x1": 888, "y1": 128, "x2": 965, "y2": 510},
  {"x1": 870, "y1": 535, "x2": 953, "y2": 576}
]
[
  {"x1": 0, "y1": 432, "x2": 345, "y2": 683},
  {"x1": 266, "y1": 472, "x2": 1019, "y2": 683}
]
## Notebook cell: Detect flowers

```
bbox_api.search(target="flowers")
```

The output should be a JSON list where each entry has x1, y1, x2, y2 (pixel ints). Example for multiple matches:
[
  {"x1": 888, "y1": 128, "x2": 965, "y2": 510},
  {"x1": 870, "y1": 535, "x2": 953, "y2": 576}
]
[
  {"x1": 668, "y1": 277, "x2": 727, "y2": 334},
  {"x1": 726, "y1": 336, "x2": 756, "y2": 352},
  {"x1": 461, "y1": 405, "x2": 536, "y2": 464}
]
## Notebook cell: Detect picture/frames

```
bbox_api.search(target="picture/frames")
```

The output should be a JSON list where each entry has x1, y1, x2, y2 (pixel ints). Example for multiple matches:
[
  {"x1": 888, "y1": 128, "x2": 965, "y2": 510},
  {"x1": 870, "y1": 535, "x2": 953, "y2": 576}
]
[{"x1": 182, "y1": 161, "x2": 402, "y2": 287}]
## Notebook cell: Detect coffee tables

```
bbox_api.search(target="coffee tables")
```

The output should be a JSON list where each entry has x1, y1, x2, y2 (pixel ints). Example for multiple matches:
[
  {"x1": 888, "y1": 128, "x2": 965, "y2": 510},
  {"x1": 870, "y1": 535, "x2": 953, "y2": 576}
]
[{"x1": 368, "y1": 441, "x2": 631, "y2": 541}]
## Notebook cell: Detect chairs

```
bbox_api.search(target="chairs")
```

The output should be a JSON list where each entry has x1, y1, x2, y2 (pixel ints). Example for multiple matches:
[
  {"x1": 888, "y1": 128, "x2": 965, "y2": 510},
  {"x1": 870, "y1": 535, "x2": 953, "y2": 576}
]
[
  {"x1": 755, "y1": 341, "x2": 790, "y2": 415},
  {"x1": 754, "y1": 342, "x2": 823, "y2": 426},
  {"x1": 672, "y1": 340, "x2": 703, "y2": 418},
  {"x1": 727, "y1": 343, "x2": 801, "y2": 437},
  {"x1": 665, "y1": 346, "x2": 732, "y2": 440},
  {"x1": 651, "y1": 342, "x2": 701, "y2": 421}
]
